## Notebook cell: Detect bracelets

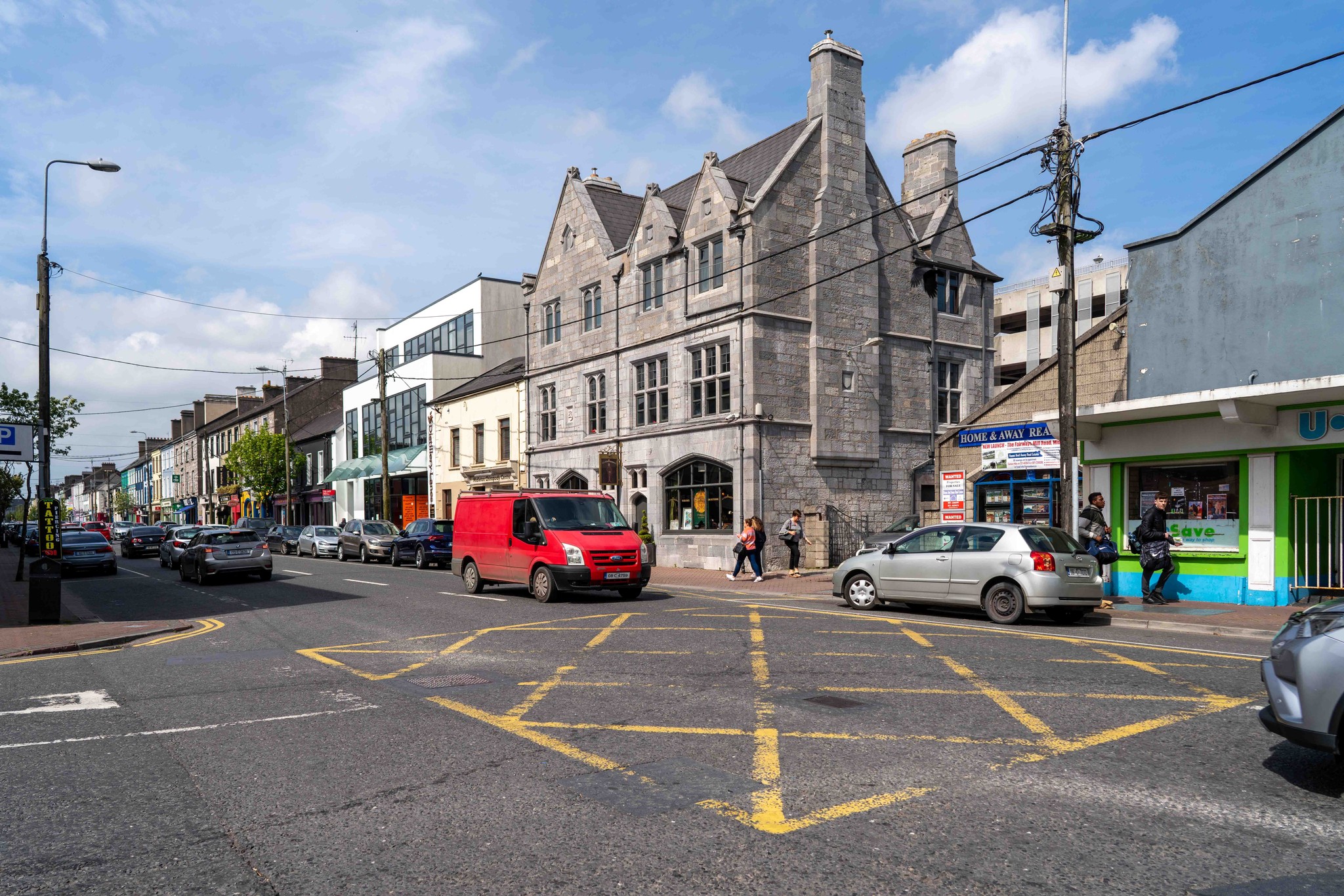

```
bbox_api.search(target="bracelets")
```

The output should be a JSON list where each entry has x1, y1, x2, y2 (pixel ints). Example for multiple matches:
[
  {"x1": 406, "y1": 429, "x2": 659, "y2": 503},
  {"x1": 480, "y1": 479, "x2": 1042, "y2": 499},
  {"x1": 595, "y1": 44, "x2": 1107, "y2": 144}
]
[{"x1": 806, "y1": 539, "x2": 808, "y2": 542}]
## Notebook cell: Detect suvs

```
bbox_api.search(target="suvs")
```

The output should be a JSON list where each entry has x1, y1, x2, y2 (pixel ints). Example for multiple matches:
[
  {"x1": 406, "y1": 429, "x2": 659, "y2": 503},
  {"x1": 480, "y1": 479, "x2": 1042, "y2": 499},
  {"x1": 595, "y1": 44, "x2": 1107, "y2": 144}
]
[
  {"x1": 337, "y1": 519, "x2": 400, "y2": 564},
  {"x1": 389, "y1": 517, "x2": 454, "y2": 570},
  {"x1": 265, "y1": 524, "x2": 306, "y2": 555},
  {"x1": 235, "y1": 516, "x2": 279, "y2": 542}
]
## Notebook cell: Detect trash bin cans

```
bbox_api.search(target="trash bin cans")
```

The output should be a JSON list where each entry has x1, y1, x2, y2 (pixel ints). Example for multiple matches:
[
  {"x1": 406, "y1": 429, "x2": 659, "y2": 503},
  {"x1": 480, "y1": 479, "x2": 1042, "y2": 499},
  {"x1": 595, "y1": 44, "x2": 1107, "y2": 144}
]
[
  {"x1": 0, "y1": 533, "x2": 8, "y2": 548},
  {"x1": 30, "y1": 556, "x2": 62, "y2": 624}
]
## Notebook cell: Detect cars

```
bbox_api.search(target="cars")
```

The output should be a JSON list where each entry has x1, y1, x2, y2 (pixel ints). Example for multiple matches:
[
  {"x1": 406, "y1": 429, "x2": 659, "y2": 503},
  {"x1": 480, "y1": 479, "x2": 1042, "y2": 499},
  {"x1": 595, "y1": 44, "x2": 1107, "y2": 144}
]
[
  {"x1": 297, "y1": 525, "x2": 343, "y2": 558},
  {"x1": 109, "y1": 521, "x2": 273, "y2": 586},
  {"x1": 2, "y1": 521, "x2": 118, "y2": 576},
  {"x1": 1258, "y1": 595, "x2": 1344, "y2": 768},
  {"x1": 831, "y1": 521, "x2": 1105, "y2": 625},
  {"x1": 859, "y1": 513, "x2": 921, "y2": 552}
]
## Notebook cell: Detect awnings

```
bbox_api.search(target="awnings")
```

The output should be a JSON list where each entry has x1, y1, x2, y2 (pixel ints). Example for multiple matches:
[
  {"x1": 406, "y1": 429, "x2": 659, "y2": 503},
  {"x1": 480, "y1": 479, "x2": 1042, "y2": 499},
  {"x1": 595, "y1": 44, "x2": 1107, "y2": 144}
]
[
  {"x1": 205, "y1": 504, "x2": 227, "y2": 514},
  {"x1": 173, "y1": 505, "x2": 196, "y2": 514},
  {"x1": 140, "y1": 512, "x2": 160, "y2": 516},
  {"x1": 77, "y1": 514, "x2": 92, "y2": 517}
]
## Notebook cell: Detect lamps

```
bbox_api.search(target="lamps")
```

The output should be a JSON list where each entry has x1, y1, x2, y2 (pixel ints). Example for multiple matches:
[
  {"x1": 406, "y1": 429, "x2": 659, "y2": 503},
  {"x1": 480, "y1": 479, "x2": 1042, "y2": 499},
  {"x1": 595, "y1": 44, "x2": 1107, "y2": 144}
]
[{"x1": 474, "y1": 472, "x2": 494, "y2": 477}]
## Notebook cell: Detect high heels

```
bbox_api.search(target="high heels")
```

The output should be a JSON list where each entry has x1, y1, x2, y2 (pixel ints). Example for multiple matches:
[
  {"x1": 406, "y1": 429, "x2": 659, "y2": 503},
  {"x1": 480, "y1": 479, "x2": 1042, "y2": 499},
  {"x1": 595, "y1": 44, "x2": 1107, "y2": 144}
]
[{"x1": 789, "y1": 572, "x2": 802, "y2": 578}]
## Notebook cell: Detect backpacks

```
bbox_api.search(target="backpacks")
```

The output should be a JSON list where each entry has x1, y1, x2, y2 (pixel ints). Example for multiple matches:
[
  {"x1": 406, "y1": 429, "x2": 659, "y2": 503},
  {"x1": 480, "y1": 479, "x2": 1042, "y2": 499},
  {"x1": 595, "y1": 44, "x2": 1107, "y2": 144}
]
[{"x1": 1128, "y1": 525, "x2": 1143, "y2": 555}]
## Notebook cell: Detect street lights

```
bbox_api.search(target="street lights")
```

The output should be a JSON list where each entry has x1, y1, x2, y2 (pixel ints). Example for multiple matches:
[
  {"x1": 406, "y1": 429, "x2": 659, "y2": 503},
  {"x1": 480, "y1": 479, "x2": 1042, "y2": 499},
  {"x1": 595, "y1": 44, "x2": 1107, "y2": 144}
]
[
  {"x1": 256, "y1": 366, "x2": 292, "y2": 526},
  {"x1": 84, "y1": 466, "x2": 97, "y2": 521},
  {"x1": 130, "y1": 430, "x2": 152, "y2": 526},
  {"x1": 40, "y1": 159, "x2": 123, "y2": 556}
]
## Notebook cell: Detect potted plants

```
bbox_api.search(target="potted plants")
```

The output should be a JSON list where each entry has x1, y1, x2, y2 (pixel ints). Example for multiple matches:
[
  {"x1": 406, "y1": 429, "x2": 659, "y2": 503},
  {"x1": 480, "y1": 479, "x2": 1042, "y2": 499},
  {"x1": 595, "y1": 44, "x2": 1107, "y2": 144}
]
[{"x1": 637, "y1": 510, "x2": 656, "y2": 566}]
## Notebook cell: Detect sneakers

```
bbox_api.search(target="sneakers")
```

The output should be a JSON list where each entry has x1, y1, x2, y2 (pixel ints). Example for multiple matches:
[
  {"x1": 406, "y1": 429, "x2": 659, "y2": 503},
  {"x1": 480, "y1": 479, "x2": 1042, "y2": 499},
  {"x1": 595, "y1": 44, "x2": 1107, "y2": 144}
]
[
  {"x1": 754, "y1": 576, "x2": 762, "y2": 582},
  {"x1": 1149, "y1": 589, "x2": 1169, "y2": 604},
  {"x1": 1142, "y1": 597, "x2": 1162, "y2": 605},
  {"x1": 726, "y1": 573, "x2": 735, "y2": 581}
]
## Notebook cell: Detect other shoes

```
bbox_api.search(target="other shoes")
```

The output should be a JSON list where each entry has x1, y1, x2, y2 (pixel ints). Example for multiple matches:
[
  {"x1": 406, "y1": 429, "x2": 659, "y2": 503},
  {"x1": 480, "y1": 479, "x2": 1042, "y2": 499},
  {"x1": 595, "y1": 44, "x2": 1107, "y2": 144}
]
[
  {"x1": 750, "y1": 572, "x2": 757, "y2": 577},
  {"x1": 754, "y1": 576, "x2": 763, "y2": 580}
]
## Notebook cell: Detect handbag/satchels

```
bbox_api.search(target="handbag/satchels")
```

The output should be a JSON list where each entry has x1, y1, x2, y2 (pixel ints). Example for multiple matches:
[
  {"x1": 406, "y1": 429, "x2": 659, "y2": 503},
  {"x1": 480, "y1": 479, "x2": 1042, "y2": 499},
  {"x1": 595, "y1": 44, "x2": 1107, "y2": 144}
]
[
  {"x1": 733, "y1": 542, "x2": 746, "y2": 561},
  {"x1": 778, "y1": 519, "x2": 796, "y2": 540},
  {"x1": 1099, "y1": 535, "x2": 1120, "y2": 565},
  {"x1": 1139, "y1": 541, "x2": 1171, "y2": 571}
]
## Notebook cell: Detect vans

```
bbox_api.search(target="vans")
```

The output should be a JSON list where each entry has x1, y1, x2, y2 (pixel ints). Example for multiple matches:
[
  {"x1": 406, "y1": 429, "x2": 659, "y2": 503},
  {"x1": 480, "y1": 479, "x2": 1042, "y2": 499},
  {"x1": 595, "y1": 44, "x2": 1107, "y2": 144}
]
[{"x1": 450, "y1": 486, "x2": 651, "y2": 603}]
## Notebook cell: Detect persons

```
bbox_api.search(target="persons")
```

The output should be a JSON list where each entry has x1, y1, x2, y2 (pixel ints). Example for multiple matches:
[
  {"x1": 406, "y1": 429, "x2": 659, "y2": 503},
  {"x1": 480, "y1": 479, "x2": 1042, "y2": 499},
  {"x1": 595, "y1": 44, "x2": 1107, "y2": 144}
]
[
  {"x1": 197, "y1": 520, "x2": 202, "y2": 525},
  {"x1": 726, "y1": 516, "x2": 766, "y2": 582},
  {"x1": 1140, "y1": 492, "x2": 1183, "y2": 604},
  {"x1": 338, "y1": 518, "x2": 347, "y2": 529},
  {"x1": 530, "y1": 504, "x2": 556, "y2": 523},
  {"x1": 779, "y1": 509, "x2": 812, "y2": 578},
  {"x1": 1212, "y1": 500, "x2": 1224, "y2": 519},
  {"x1": 1078, "y1": 492, "x2": 1112, "y2": 577}
]
